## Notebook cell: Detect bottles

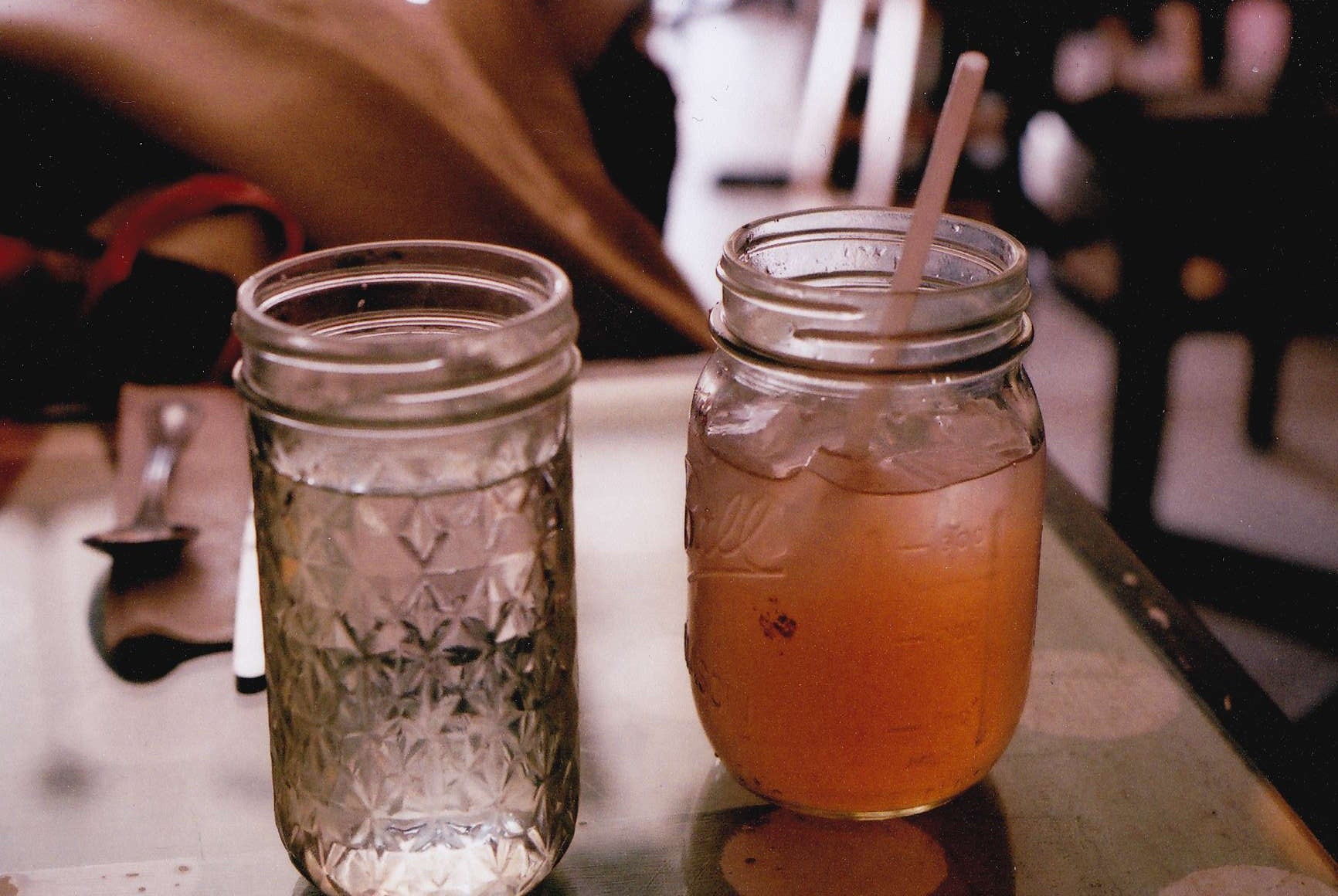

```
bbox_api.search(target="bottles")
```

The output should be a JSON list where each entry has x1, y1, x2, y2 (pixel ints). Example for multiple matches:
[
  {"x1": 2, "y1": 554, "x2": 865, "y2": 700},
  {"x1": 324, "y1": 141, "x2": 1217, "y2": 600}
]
[
  {"x1": 678, "y1": 203, "x2": 1054, "y2": 820},
  {"x1": 231, "y1": 239, "x2": 583, "y2": 896}
]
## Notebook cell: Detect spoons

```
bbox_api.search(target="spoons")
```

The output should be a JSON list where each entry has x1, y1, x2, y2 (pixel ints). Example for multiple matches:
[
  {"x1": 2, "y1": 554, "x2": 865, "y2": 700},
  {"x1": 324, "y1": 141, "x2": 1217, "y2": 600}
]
[{"x1": 81, "y1": 397, "x2": 202, "y2": 554}]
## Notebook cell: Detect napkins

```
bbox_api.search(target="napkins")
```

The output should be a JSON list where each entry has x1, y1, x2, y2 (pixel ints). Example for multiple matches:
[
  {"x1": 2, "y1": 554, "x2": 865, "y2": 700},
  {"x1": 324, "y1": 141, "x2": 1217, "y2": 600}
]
[{"x1": 107, "y1": 383, "x2": 250, "y2": 648}]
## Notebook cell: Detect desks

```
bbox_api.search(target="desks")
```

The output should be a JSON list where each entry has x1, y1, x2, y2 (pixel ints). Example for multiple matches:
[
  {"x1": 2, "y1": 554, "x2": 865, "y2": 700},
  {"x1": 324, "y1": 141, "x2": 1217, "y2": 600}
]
[{"x1": 2, "y1": 348, "x2": 1338, "y2": 895}]
